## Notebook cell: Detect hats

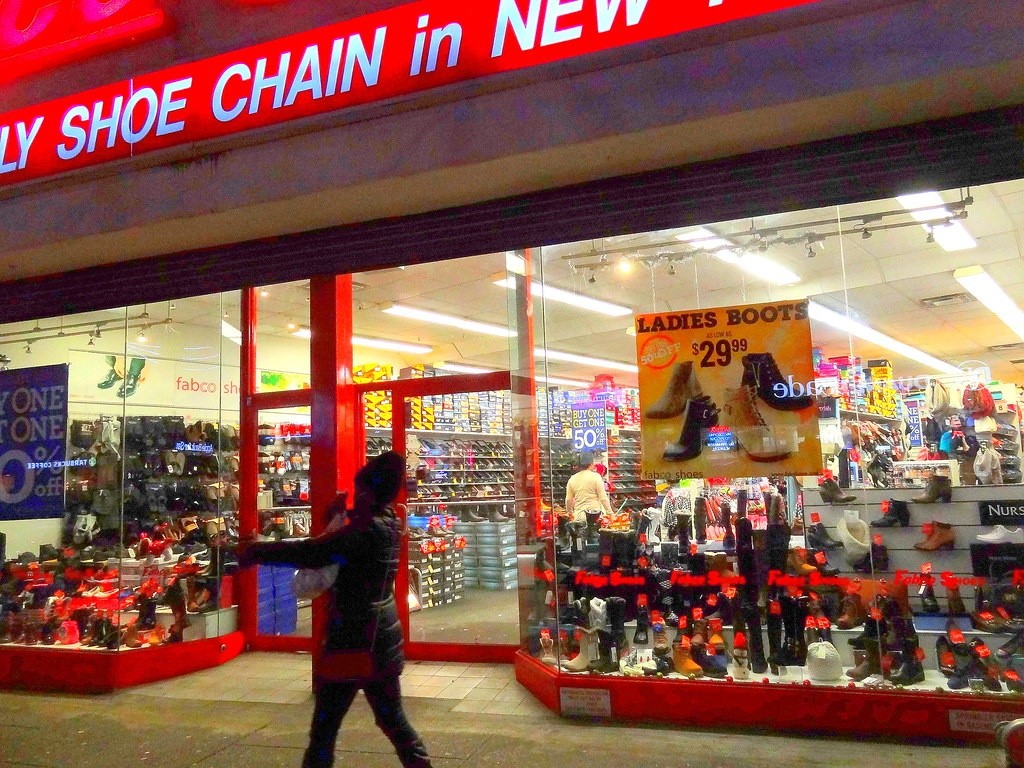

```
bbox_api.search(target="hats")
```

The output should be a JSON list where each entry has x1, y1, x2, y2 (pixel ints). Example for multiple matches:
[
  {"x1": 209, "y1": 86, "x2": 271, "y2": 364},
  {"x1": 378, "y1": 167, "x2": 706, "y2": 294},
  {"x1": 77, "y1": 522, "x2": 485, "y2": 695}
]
[{"x1": 354, "y1": 451, "x2": 404, "y2": 502}]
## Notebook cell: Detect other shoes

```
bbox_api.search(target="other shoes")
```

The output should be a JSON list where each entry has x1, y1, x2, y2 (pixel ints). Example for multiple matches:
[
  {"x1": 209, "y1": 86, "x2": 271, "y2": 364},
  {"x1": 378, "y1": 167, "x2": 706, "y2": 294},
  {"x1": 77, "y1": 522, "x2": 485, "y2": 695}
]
[
  {"x1": 4, "y1": 413, "x2": 246, "y2": 651},
  {"x1": 365, "y1": 429, "x2": 642, "y2": 522},
  {"x1": 408, "y1": 525, "x2": 456, "y2": 540}
]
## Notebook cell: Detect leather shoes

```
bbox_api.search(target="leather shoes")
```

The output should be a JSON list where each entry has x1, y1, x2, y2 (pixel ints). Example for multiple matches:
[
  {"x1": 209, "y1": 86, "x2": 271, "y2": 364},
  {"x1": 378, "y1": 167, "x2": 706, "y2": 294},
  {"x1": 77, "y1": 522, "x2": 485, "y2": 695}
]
[
  {"x1": 97, "y1": 370, "x2": 118, "y2": 387},
  {"x1": 117, "y1": 374, "x2": 144, "y2": 397}
]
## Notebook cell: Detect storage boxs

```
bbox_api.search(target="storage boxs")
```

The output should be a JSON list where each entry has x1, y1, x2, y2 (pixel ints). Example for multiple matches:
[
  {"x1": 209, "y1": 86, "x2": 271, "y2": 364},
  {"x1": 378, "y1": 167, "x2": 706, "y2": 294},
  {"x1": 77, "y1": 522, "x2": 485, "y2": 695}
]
[
  {"x1": 406, "y1": 537, "x2": 464, "y2": 607},
  {"x1": 223, "y1": 557, "x2": 296, "y2": 639},
  {"x1": 339, "y1": 363, "x2": 641, "y2": 438},
  {"x1": 811, "y1": 348, "x2": 899, "y2": 420},
  {"x1": 448, "y1": 520, "x2": 515, "y2": 592}
]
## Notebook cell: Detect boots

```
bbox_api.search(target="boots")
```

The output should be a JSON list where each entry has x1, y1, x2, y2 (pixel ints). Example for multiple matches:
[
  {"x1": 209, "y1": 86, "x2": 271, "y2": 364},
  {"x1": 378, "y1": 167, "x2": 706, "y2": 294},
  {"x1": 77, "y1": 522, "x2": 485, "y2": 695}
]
[
  {"x1": 721, "y1": 385, "x2": 793, "y2": 462},
  {"x1": 527, "y1": 451, "x2": 1023, "y2": 692},
  {"x1": 741, "y1": 352, "x2": 811, "y2": 411},
  {"x1": 646, "y1": 361, "x2": 706, "y2": 419},
  {"x1": 665, "y1": 395, "x2": 720, "y2": 465}
]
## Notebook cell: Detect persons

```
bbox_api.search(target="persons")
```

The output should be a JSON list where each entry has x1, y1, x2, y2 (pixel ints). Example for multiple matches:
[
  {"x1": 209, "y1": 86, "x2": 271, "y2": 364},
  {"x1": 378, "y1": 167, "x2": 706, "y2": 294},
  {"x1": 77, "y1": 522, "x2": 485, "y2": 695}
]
[
  {"x1": 565, "y1": 452, "x2": 617, "y2": 526},
  {"x1": 235, "y1": 450, "x2": 433, "y2": 768}
]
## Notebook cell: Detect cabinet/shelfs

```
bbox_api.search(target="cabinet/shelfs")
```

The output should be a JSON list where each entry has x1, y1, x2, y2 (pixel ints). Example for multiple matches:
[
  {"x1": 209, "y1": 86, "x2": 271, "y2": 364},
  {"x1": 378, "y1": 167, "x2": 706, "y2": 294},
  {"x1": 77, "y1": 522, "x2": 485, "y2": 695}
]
[
  {"x1": 0, "y1": 553, "x2": 237, "y2": 651},
  {"x1": 532, "y1": 485, "x2": 1021, "y2": 694},
  {"x1": 64, "y1": 420, "x2": 251, "y2": 548},
  {"x1": 257, "y1": 422, "x2": 657, "y2": 539},
  {"x1": 815, "y1": 379, "x2": 1022, "y2": 485}
]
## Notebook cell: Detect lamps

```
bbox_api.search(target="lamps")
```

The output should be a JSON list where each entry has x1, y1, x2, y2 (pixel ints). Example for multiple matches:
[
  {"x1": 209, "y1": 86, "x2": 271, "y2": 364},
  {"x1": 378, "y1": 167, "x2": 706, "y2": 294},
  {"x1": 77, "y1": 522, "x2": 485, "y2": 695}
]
[
  {"x1": 0, "y1": 301, "x2": 171, "y2": 360},
  {"x1": 561, "y1": 185, "x2": 973, "y2": 284}
]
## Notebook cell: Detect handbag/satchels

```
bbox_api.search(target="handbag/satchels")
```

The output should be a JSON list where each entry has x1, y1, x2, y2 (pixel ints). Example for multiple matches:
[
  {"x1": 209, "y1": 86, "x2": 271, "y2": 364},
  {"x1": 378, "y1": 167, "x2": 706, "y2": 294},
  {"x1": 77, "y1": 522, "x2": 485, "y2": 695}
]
[
  {"x1": 291, "y1": 510, "x2": 349, "y2": 599},
  {"x1": 311, "y1": 647, "x2": 382, "y2": 693}
]
